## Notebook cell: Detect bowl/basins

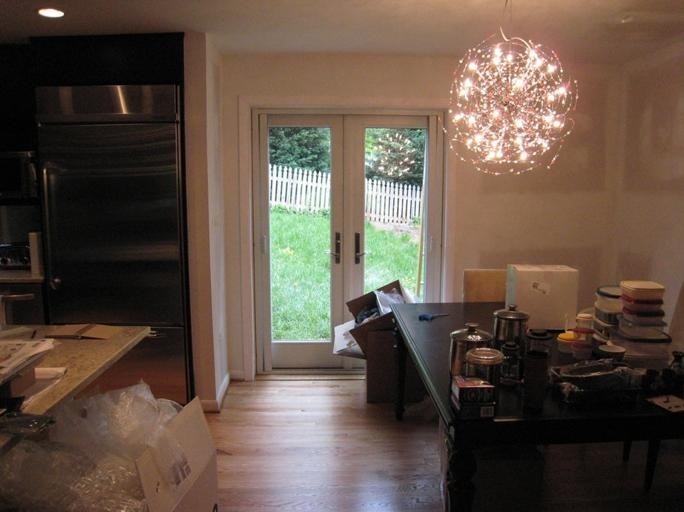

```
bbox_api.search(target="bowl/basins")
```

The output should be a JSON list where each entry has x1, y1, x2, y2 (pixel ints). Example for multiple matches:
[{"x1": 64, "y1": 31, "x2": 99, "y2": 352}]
[
  {"x1": 597, "y1": 344, "x2": 626, "y2": 367},
  {"x1": 574, "y1": 326, "x2": 593, "y2": 341},
  {"x1": 557, "y1": 333, "x2": 576, "y2": 353},
  {"x1": 570, "y1": 343, "x2": 592, "y2": 359},
  {"x1": 575, "y1": 314, "x2": 592, "y2": 330}
]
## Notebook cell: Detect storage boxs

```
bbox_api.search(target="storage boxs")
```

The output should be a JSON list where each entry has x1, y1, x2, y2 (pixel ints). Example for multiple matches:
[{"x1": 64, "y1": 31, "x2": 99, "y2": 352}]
[
  {"x1": 346, "y1": 279, "x2": 414, "y2": 356},
  {"x1": 505, "y1": 264, "x2": 579, "y2": 328},
  {"x1": 135, "y1": 396, "x2": 218, "y2": 511},
  {"x1": 333, "y1": 330, "x2": 429, "y2": 403}
]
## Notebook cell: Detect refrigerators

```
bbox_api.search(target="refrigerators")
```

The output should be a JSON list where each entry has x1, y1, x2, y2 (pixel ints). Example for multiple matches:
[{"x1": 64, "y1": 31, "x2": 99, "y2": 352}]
[{"x1": 34, "y1": 84, "x2": 187, "y2": 406}]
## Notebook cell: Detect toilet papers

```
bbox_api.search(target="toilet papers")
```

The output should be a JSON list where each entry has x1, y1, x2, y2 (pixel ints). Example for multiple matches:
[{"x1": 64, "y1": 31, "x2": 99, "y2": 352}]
[{"x1": 29, "y1": 232, "x2": 42, "y2": 277}]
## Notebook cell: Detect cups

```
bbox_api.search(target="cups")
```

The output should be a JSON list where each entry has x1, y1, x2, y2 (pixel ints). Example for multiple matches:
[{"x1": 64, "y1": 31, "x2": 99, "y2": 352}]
[
  {"x1": 493, "y1": 304, "x2": 529, "y2": 350},
  {"x1": 448, "y1": 323, "x2": 495, "y2": 378}
]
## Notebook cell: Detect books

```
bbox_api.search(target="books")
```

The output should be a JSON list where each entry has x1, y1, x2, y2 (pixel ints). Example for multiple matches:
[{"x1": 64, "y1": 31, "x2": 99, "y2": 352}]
[{"x1": 44, "y1": 324, "x2": 116, "y2": 340}]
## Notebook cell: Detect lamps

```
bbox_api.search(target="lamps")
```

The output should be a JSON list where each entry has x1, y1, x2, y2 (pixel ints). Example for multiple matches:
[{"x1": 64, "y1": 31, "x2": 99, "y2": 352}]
[{"x1": 442, "y1": 1, "x2": 579, "y2": 176}]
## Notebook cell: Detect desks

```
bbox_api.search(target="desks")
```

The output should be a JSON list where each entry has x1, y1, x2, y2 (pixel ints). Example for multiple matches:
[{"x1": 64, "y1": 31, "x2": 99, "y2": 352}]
[
  {"x1": 391, "y1": 304, "x2": 684, "y2": 512},
  {"x1": 1, "y1": 323, "x2": 150, "y2": 455}
]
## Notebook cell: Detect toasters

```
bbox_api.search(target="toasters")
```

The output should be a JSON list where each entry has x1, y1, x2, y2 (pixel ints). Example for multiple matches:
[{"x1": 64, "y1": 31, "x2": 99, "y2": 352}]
[{"x1": 0, "y1": 241, "x2": 30, "y2": 269}]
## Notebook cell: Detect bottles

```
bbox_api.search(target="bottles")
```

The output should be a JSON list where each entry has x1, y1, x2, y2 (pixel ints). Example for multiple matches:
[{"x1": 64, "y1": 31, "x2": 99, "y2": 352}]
[
  {"x1": 466, "y1": 347, "x2": 503, "y2": 405},
  {"x1": 498, "y1": 342, "x2": 522, "y2": 386},
  {"x1": 670, "y1": 350, "x2": 684, "y2": 391},
  {"x1": 527, "y1": 330, "x2": 553, "y2": 357}
]
